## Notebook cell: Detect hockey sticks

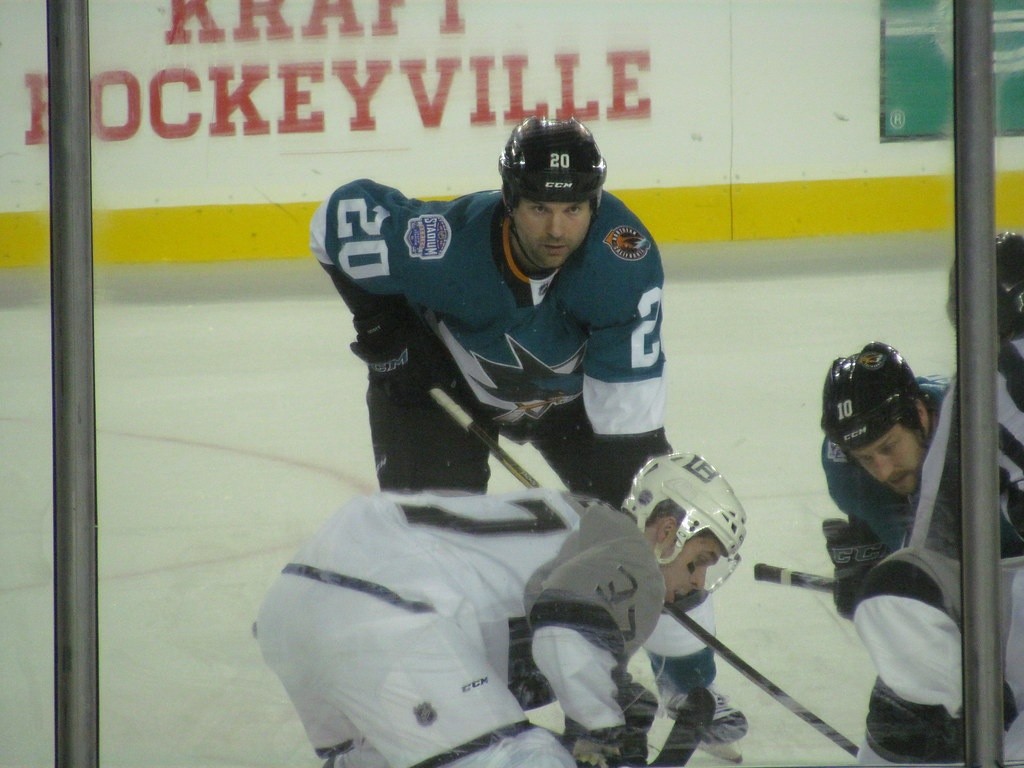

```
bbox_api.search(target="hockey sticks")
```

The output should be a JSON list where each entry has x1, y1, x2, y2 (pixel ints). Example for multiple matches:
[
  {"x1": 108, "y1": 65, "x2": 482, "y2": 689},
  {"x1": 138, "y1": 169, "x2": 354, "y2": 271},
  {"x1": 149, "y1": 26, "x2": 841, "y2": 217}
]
[
  {"x1": 526, "y1": 686, "x2": 720, "y2": 768},
  {"x1": 753, "y1": 563, "x2": 833, "y2": 592},
  {"x1": 430, "y1": 386, "x2": 861, "y2": 757}
]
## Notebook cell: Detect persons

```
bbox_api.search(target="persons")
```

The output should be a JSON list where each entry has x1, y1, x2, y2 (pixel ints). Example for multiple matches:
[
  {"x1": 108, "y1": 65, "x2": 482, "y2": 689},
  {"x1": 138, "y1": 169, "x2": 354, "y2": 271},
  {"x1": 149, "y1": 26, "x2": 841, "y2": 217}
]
[
  {"x1": 309, "y1": 115, "x2": 749, "y2": 766},
  {"x1": 819, "y1": 338, "x2": 965, "y2": 621},
  {"x1": 853, "y1": 230, "x2": 1024, "y2": 768},
  {"x1": 254, "y1": 450, "x2": 749, "y2": 768}
]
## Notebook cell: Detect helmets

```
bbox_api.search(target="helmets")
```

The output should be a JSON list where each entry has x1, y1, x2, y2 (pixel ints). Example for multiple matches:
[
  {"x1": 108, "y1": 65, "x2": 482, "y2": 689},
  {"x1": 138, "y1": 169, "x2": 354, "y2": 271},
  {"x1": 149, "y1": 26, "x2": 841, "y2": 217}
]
[
  {"x1": 819, "y1": 341, "x2": 920, "y2": 450},
  {"x1": 621, "y1": 450, "x2": 748, "y2": 563},
  {"x1": 498, "y1": 115, "x2": 607, "y2": 202},
  {"x1": 947, "y1": 230, "x2": 1024, "y2": 340}
]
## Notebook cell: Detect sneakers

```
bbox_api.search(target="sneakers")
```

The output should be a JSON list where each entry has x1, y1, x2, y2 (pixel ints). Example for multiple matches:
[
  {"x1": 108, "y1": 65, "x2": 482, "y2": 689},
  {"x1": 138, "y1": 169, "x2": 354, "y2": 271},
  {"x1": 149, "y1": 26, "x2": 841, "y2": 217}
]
[{"x1": 647, "y1": 654, "x2": 748, "y2": 763}]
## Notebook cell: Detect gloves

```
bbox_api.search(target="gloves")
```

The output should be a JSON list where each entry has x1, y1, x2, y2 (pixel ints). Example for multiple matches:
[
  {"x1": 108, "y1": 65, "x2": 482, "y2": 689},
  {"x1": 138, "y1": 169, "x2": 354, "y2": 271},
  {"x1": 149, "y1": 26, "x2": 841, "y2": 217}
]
[
  {"x1": 351, "y1": 298, "x2": 462, "y2": 409},
  {"x1": 572, "y1": 734, "x2": 623, "y2": 768}
]
